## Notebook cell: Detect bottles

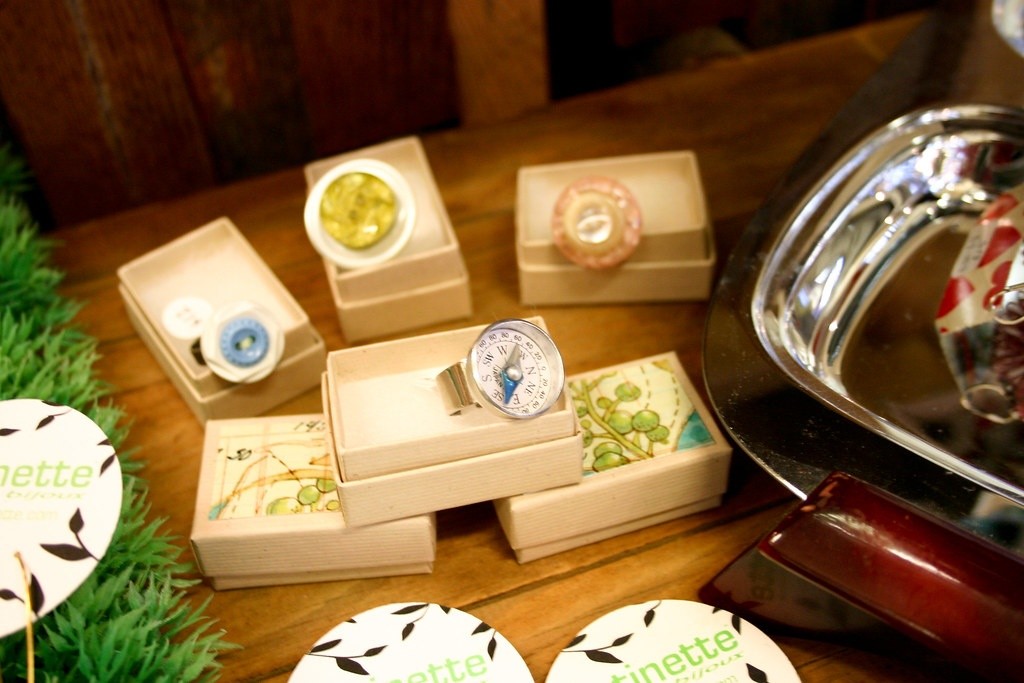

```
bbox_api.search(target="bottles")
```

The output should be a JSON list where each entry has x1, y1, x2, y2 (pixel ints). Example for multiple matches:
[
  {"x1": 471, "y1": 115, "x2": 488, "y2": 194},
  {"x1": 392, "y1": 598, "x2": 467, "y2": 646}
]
[{"x1": 933, "y1": 180, "x2": 1024, "y2": 424}]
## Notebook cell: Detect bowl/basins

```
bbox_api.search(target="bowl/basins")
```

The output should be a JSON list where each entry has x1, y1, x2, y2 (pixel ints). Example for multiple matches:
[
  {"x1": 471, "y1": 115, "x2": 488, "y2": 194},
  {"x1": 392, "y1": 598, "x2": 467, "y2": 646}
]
[{"x1": 751, "y1": 103, "x2": 1024, "y2": 510}]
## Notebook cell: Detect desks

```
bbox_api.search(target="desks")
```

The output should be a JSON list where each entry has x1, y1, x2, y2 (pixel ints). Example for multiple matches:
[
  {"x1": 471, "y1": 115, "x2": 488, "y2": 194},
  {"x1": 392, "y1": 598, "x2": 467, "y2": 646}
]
[{"x1": 37, "y1": 0, "x2": 1024, "y2": 683}]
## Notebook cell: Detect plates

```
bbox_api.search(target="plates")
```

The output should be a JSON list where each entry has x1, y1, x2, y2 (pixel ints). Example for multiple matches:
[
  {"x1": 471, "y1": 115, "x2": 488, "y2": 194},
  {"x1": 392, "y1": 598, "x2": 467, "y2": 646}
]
[
  {"x1": 287, "y1": 601, "x2": 535, "y2": 683},
  {"x1": 703, "y1": 99, "x2": 1024, "y2": 558},
  {"x1": 991, "y1": 0, "x2": 1024, "y2": 58},
  {"x1": 545, "y1": 598, "x2": 802, "y2": 682},
  {"x1": 1, "y1": 399, "x2": 124, "y2": 640}
]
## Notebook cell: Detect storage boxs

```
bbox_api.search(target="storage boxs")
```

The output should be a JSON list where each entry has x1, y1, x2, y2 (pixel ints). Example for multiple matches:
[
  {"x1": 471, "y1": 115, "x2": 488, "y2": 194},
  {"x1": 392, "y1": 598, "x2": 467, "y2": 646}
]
[{"x1": 119, "y1": 135, "x2": 735, "y2": 591}]
